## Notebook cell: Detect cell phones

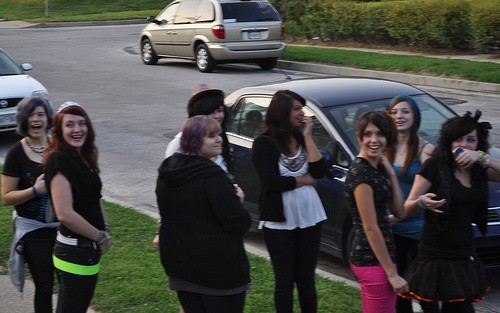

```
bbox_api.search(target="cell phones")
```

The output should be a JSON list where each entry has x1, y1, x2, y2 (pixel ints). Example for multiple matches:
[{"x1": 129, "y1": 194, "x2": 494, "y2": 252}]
[{"x1": 454, "y1": 147, "x2": 474, "y2": 171}]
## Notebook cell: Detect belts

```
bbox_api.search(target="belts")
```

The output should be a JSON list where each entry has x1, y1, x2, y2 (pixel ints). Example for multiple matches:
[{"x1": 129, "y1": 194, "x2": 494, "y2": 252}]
[{"x1": 56, "y1": 230, "x2": 98, "y2": 249}]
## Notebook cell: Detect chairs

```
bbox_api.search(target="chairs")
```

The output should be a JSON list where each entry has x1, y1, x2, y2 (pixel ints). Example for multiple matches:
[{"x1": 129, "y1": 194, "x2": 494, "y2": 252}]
[{"x1": 238, "y1": 111, "x2": 262, "y2": 138}]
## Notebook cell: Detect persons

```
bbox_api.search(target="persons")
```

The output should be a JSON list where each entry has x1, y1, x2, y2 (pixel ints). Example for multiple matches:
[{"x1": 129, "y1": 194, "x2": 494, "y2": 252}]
[
  {"x1": 153, "y1": 88, "x2": 234, "y2": 313},
  {"x1": 344, "y1": 111, "x2": 410, "y2": 313},
  {"x1": 42, "y1": 101, "x2": 113, "y2": 313},
  {"x1": 403, "y1": 109, "x2": 500, "y2": 313},
  {"x1": 1, "y1": 92, "x2": 59, "y2": 313},
  {"x1": 155, "y1": 115, "x2": 252, "y2": 313},
  {"x1": 252, "y1": 90, "x2": 329, "y2": 313},
  {"x1": 381, "y1": 94, "x2": 436, "y2": 313}
]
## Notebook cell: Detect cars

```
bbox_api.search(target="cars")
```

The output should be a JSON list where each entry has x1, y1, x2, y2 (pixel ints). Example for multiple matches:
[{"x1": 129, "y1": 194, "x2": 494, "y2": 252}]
[
  {"x1": 137, "y1": 0, "x2": 287, "y2": 70},
  {"x1": 165, "y1": 75, "x2": 500, "y2": 281},
  {"x1": 0, "y1": 47, "x2": 54, "y2": 134}
]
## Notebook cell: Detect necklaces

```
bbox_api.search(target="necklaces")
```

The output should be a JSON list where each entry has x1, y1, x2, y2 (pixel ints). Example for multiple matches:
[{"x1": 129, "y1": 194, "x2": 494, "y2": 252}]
[{"x1": 26, "y1": 135, "x2": 51, "y2": 153}]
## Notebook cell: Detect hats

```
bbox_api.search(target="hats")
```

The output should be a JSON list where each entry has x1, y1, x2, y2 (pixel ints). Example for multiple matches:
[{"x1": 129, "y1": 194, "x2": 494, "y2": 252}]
[
  {"x1": 441, "y1": 114, "x2": 476, "y2": 140},
  {"x1": 189, "y1": 89, "x2": 224, "y2": 117}
]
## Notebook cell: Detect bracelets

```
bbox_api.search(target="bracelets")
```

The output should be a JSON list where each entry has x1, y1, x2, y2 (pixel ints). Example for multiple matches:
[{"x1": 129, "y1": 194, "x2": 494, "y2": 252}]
[
  {"x1": 96, "y1": 232, "x2": 106, "y2": 246},
  {"x1": 477, "y1": 151, "x2": 488, "y2": 164},
  {"x1": 418, "y1": 200, "x2": 425, "y2": 209},
  {"x1": 32, "y1": 186, "x2": 40, "y2": 197}
]
